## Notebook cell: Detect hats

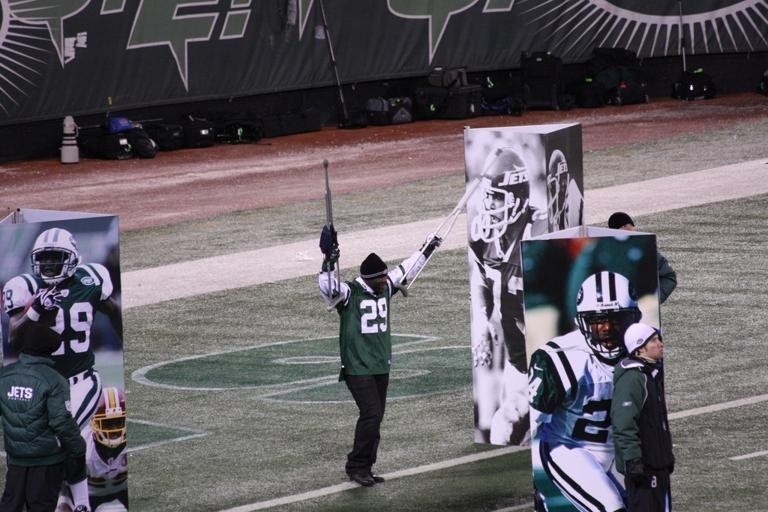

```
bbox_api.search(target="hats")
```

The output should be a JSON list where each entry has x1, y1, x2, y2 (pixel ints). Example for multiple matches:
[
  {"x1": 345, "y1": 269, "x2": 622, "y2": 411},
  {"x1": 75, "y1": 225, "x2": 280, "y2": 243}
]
[
  {"x1": 20, "y1": 324, "x2": 61, "y2": 355},
  {"x1": 625, "y1": 323, "x2": 655, "y2": 354},
  {"x1": 361, "y1": 253, "x2": 388, "y2": 279}
]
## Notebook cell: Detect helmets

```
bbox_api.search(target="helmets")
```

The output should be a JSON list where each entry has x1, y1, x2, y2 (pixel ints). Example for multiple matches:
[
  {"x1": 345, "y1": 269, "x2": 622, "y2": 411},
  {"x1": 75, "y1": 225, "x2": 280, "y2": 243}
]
[
  {"x1": 577, "y1": 271, "x2": 638, "y2": 364},
  {"x1": 91, "y1": 387, "x2": 125, "y2": 449},
  {"x1": 30, "y1": 228, "x2": 79, "y2": 283},
  {"x1": 543, "y1": 150, "x2": 569, "y2": 225},
  {"x1": 480, "y1": 149, "x2": 531, "y2": 243}
]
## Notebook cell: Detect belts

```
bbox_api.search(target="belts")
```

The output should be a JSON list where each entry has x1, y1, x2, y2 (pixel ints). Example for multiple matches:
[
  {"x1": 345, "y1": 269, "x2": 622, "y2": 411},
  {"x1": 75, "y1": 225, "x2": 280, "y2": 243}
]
[{"x1": 322, "y1": 249, "x2": 340, "y2": 271}]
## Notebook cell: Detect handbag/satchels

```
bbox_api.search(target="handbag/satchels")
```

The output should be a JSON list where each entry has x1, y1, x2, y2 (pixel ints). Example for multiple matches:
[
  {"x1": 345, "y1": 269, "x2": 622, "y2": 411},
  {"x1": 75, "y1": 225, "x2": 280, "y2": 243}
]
[
  {"x1": 363, "y1": 65, "x2": 482, "y2": 125},
  {"x1": 77, "y1": 112, "x2": 263, "y2": 160},
  {"x1": 483, "y1": 49, "x2": 648, "y2": 116},
  {"x1": 671, "y1": 67, "x2": 715, "y2": 101}
]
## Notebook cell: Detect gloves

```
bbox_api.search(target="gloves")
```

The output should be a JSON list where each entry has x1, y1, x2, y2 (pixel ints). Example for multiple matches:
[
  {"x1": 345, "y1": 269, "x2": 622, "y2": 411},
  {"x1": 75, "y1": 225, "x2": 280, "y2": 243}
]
[
  {"x1": 27, "y1": 286, "x2": 62, "y2": 321},
  {"x1": 626, "y1": 459, "x2": 650, "y2": 487},
  {"x1": 420, "y1": 236, "x2": 441, "y2": 259},
  {"x1": 73, "y1": 454, "x2": 86, "y2": 469}
]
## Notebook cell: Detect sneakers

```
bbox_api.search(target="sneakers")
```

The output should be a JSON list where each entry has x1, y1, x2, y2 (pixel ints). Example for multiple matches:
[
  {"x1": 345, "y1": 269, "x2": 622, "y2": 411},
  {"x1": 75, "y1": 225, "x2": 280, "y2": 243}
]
[{"x1": 351, "y1": 470, "x2": 385, "y2": 486}]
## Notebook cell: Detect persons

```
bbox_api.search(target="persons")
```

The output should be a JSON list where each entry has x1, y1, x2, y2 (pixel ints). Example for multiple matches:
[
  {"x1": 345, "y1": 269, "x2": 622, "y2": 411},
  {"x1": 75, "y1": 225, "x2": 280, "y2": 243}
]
[
  {"x1": 469, "y1": 153, "x2": 547, "y2": 446},
  {"x1": 0, "y1": 325, "x2": 86, "y2": 512},
  {"x1": 607, "y1": 324, "x2": 676, "y2": 512},
  {"x1": 530, "y1": 266, "x2": 643, "y2": 512},
  {"x1": 604, "y1": 214, "x2": 679, "y2": 308},
  {"x1": 547, "y1": 145, "x2": 585, "y2": 235},
  {"x1": 61, "y1": 384, "x2": 130, "y2": 511},
  {"x1": 317, "y1": 221, "x2": 443, "y2": 486},
  {"x1": 2, "y1": 228, "x2": 124, "y2": 511}
]
